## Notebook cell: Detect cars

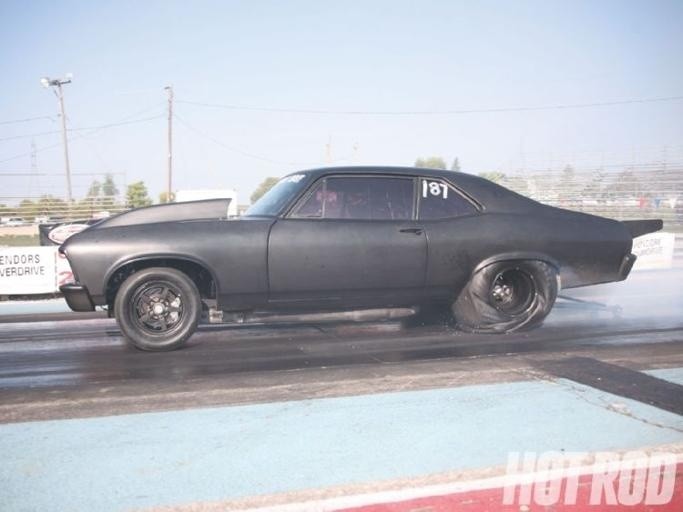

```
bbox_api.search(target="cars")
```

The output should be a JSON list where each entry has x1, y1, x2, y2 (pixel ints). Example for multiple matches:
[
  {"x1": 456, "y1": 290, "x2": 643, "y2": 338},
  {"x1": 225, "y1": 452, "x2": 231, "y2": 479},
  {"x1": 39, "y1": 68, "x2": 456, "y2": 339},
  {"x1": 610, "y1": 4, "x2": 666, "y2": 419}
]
[{"x1": 6, "y1": 216, "x2": 26, "y2": 226}]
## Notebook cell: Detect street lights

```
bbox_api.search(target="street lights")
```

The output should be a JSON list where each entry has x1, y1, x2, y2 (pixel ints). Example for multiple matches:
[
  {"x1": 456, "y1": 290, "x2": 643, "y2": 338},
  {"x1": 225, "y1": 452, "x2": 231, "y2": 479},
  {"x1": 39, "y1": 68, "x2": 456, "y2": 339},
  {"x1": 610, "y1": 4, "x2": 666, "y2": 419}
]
[
  {"x1": 161, "y1": 84, "x2": 175, "y2": 201},
  {"x1": 40, "y1": 73, "x2": 73, "y2": 217}
]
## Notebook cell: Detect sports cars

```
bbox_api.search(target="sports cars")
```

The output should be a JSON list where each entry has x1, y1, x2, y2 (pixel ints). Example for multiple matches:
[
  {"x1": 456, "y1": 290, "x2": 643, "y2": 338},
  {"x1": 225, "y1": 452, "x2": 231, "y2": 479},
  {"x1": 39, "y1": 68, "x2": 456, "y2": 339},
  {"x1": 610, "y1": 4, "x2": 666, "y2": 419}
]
[{"x1": 54, "y1": 166, "x2": 664, "y2": 355}]
